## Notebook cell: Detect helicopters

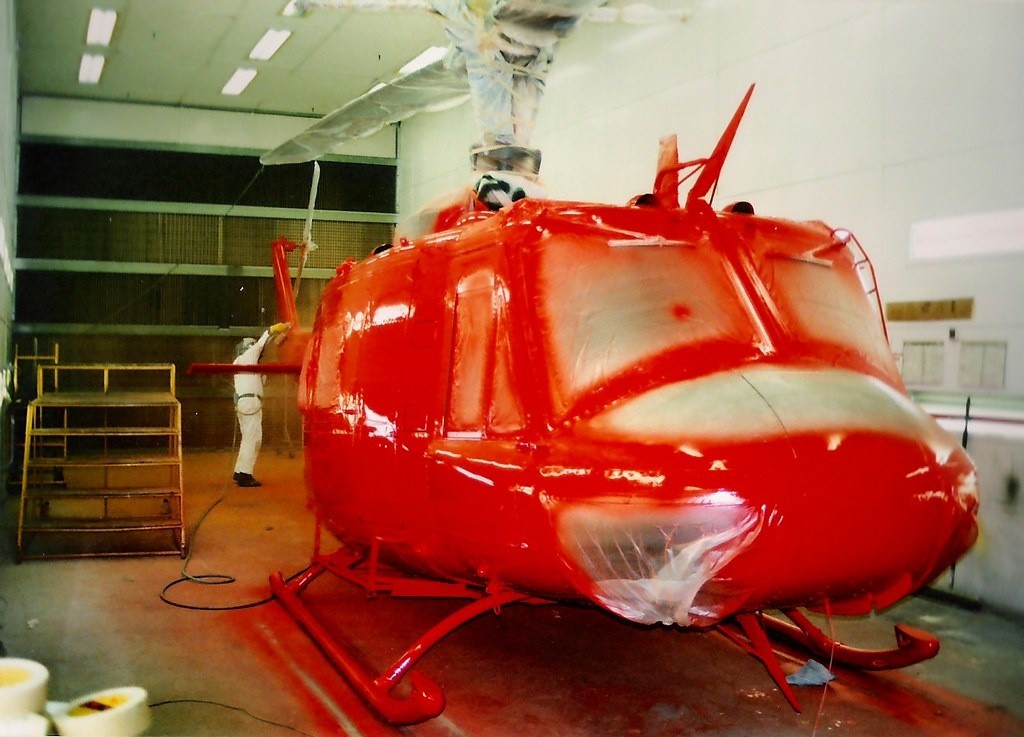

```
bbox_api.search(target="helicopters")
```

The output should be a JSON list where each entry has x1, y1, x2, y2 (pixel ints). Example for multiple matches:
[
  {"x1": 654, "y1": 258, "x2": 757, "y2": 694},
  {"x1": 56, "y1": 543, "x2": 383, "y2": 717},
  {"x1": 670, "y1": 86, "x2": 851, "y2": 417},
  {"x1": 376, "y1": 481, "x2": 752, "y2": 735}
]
[{"x1": 180, "y1": 1, "x2": 984, "y2": 729}]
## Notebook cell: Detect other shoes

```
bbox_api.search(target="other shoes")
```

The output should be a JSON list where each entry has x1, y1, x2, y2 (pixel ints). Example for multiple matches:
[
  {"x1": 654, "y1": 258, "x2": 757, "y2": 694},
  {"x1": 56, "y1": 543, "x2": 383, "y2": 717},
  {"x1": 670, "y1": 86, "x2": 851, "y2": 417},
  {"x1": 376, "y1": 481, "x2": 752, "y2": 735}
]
[{"x1": 234, "y1": 470, "x2": 262, "y2": 486}]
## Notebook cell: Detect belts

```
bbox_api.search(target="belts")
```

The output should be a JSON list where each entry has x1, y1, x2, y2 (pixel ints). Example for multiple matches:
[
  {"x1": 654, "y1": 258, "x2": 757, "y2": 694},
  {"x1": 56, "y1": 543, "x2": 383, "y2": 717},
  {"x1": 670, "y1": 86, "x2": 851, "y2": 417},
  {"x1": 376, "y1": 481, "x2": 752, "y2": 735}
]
[{"x1": 239, "y1": 394, "x2": 261, "y2": 399}]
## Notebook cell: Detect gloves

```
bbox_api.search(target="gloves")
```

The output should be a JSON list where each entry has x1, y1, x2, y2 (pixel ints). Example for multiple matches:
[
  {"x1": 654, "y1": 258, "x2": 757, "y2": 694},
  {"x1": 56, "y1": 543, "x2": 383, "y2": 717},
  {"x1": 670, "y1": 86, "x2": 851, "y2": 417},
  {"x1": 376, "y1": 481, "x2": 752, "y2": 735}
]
[{"x1": 269, "y1": 323, "x2": 286, "y2": 335}]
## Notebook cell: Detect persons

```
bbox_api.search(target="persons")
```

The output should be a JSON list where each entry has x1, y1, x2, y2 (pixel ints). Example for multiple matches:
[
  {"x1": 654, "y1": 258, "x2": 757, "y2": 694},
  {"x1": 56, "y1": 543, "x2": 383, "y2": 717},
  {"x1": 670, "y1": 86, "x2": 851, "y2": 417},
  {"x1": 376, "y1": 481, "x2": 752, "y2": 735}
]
[{"x1": 232, "y1": 323, "x2": 286, "y2": 488}]
